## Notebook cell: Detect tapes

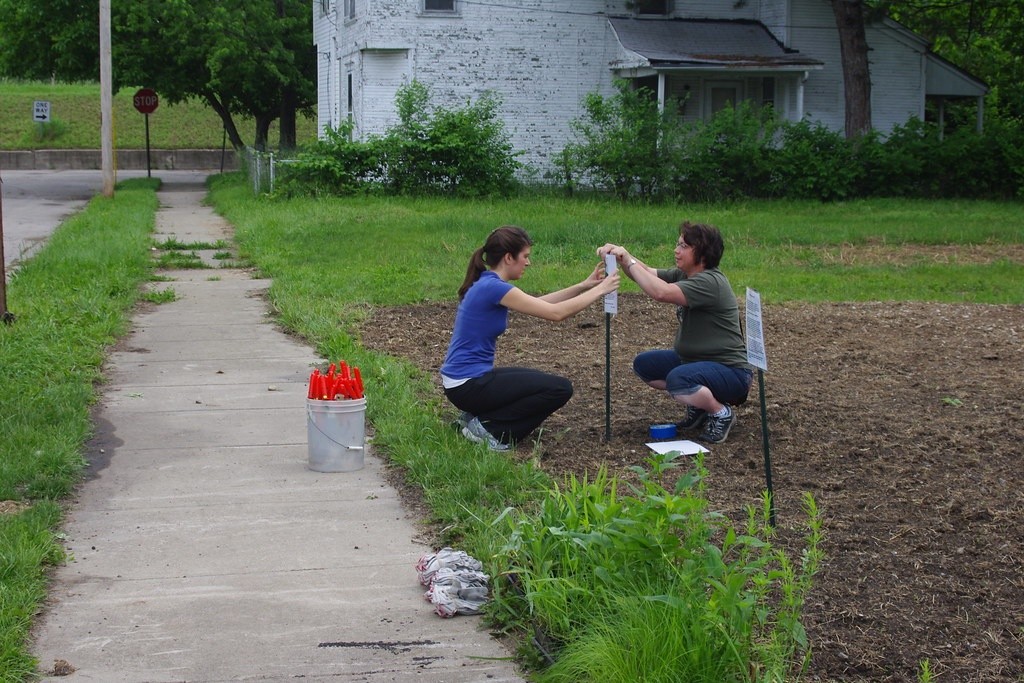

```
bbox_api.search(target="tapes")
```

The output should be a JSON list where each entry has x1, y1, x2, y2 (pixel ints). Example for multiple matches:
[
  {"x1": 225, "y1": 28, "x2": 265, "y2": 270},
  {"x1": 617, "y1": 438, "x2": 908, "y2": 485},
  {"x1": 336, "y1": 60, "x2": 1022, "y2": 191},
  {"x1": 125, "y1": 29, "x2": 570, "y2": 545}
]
[{"x1": 649, "y1": 423, "x2": 678, "y2": 440}]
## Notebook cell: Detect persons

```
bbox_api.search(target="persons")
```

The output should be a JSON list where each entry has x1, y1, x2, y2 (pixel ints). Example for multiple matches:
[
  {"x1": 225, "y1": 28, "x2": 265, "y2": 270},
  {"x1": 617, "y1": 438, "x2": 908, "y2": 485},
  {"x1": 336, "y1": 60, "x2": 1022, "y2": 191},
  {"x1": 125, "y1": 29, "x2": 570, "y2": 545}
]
[
  {"x1": 442, "y1": 226, "x2": 621, "y2": 450},
  {"x1": 596, "y1": 221, "x2": 755, "y2": 444}
]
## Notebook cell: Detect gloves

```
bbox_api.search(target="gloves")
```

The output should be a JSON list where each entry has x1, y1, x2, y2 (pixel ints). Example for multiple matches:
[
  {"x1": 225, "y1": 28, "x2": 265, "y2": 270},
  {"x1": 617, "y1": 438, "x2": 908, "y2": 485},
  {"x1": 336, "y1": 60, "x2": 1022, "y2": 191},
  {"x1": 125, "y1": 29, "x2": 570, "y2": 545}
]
[{"x1": 415, "y1": 547, "x2": 491, "y2": 618}]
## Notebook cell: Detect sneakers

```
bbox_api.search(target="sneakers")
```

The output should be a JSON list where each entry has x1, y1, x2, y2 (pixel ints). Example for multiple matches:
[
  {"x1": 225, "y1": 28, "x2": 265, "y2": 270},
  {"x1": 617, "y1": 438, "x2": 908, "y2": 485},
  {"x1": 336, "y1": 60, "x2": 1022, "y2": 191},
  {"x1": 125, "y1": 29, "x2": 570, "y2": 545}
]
[
  {"x1": 698, "y1": 404, "x2": 736, "y2": 444},
  {"x1": 675, "y1": 404, "x2": 706, "y2": 430},
  {"x1": 456, "y1": 410, "x2": 475, "y2": 427},
  {"x1": 462, "y1": 416, "x2": 510, "y2": 453}
]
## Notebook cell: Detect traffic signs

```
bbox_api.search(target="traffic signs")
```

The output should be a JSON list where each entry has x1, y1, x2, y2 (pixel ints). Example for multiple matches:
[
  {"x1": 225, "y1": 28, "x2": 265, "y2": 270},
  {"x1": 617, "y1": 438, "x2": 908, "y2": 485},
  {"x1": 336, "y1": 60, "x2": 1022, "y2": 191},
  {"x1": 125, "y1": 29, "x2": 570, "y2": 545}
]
[{"x1": 33, "y1": 101, "x2": 50, "y2": 122}]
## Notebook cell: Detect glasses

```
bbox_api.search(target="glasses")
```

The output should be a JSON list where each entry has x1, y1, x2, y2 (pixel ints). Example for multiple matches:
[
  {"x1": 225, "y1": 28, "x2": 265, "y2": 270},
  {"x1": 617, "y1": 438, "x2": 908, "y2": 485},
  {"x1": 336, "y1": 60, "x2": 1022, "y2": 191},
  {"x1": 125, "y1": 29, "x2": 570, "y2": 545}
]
[{"x1": 676, "y1": 242, "x2": 691, "y2": 249}]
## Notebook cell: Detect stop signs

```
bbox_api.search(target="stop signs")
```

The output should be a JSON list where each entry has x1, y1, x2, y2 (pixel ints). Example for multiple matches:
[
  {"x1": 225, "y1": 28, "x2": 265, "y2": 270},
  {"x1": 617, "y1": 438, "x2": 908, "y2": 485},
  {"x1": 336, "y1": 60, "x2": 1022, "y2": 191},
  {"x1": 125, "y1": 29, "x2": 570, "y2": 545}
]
[{"x1": 133, "y1": 88, "x2": 159, "y2": 114}]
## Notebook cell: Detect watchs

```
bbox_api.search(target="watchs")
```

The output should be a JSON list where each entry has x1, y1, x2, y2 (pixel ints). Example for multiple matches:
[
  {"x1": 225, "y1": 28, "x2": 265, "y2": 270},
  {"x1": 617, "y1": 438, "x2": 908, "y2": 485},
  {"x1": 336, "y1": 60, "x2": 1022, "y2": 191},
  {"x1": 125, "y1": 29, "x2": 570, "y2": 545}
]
[{"x1": 626, "y1": 258, "x2": 636, "y2": 271}]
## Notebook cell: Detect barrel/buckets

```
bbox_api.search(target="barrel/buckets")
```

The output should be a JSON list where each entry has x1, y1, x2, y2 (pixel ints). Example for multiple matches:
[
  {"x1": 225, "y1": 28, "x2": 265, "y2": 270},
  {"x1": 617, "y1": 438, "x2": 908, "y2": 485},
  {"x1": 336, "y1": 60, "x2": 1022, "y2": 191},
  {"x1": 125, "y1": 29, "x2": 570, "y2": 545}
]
[{"x1": 307, "y1": 394, "x2": 367, "y2": 472}]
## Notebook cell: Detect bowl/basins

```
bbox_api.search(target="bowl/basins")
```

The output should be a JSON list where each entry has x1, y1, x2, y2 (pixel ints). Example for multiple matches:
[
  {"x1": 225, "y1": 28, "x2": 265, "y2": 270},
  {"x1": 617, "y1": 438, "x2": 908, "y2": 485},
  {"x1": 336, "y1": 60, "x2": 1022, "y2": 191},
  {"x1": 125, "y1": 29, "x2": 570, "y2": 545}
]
[{"x1": 650, "y1": 425, "x2": 676, "y2": 439}]
